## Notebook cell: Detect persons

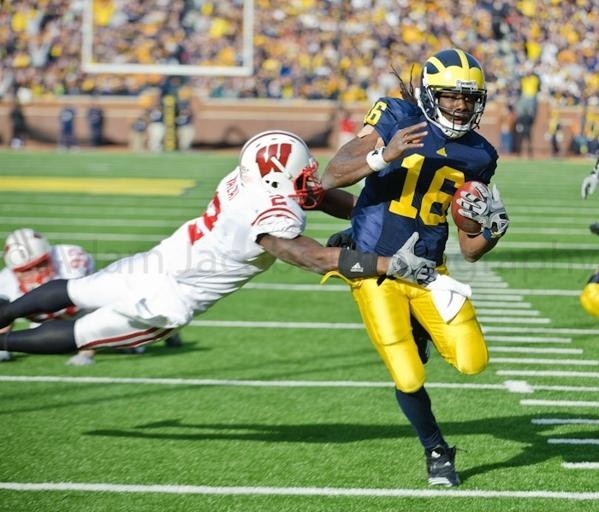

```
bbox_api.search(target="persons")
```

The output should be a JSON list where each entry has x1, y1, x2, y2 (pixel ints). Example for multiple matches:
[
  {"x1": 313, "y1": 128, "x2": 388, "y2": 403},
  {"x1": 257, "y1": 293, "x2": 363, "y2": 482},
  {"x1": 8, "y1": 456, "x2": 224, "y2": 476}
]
[
  {"x1": 579, "y1": 222, "x2": 599, "y2": 317},
  {"x1": 321, "y1": 49, "x2": 510, "y2": 488},
  {"x1": 0, "y1": 130, "x2": 437, "y2": 355},
  {"x1": 0, "y1": 227, "x2": 182, "y2": 365},
  {"x1": 580, "y1": 157, "x2": 599, "y2": 199}
]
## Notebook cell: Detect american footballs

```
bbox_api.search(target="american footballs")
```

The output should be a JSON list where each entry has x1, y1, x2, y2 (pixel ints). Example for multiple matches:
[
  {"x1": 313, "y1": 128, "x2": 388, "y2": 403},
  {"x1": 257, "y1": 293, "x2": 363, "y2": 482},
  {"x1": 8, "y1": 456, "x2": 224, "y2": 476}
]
[{"x1": 452, "y1": 181, "x2": 487, "y2": 236}]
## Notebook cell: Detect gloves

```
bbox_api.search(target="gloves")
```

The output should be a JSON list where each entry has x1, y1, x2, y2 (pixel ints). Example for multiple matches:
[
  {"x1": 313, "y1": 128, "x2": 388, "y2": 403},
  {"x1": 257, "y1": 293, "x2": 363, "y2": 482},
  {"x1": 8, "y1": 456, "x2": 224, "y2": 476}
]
[
  {"x1": 581, "y1": 174, "x2": 599, "y2": 199},
  {"x1": 455, "y1": 180, "x2": 510, "y2": 244},
  {"x1": 384, "y1": 231, "x2": 438, "y2": 287}
]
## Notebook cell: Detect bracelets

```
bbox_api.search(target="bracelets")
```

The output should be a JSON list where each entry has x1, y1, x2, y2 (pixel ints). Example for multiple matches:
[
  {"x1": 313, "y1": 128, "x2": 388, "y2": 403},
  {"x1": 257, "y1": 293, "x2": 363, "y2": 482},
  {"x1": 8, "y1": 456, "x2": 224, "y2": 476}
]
[
  {"x1": 365, "y1": 146, "x2": 392, "y2": 172},
  {"x1": 338, "y1": 248, "x2": 378, "y2": 279}
]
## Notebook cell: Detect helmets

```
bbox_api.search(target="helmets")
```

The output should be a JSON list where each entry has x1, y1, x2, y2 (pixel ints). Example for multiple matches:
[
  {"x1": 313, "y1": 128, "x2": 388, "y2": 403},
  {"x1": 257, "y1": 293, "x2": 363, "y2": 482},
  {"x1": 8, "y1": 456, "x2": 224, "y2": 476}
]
[
  {"x1": 237, "y1": 128, "x2": 324, "y2": 210},
  {"x1": 414, "y1": 48, "x2": 489, "y2": 141},
  {"x1": 2, "y1": 228, "x2": 57, "y2": 296}
]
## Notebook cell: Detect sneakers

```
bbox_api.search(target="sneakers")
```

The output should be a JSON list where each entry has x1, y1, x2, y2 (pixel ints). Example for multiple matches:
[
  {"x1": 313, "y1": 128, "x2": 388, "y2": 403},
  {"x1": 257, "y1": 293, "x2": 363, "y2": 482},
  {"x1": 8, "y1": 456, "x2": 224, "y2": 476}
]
[
  {"x1": 410, "y1": 315, "x2": 431, "y2": 365},
  {"x1": 424, "y1": 442, "x2": 462, "y2": 489}
]
